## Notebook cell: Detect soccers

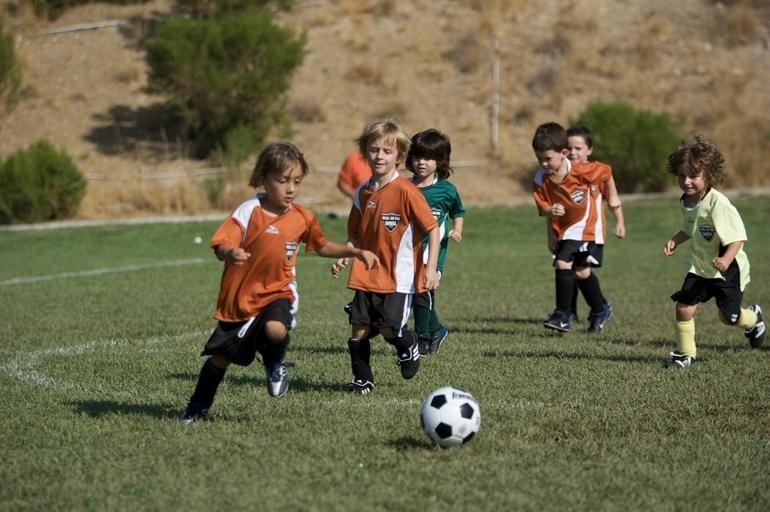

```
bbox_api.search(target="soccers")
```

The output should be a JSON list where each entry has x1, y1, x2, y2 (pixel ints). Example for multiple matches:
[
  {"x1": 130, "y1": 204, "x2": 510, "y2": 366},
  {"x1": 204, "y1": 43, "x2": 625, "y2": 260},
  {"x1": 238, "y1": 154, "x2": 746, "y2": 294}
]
[{"x1": 417, "y1": 384, "x2": 483, "y2": 446}]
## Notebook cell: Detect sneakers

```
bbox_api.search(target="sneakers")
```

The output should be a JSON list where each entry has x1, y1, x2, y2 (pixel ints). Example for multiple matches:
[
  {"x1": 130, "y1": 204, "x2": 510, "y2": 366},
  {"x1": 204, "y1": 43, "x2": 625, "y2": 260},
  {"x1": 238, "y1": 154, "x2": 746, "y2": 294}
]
[
  {"x1": 586, "y1": 304, "x2": 613, "y2": 333},
  {"x1": 345, "y1": 379, "x2": 374, "y2": 396},
  {"x1": 744, "y1": 304, "x2": 766, "y2": 349},
  {"x1": 543, "y1": 310, "x2": 571, "y2": 332},
  {"x1": 179, "y1": 402, "x2": 209, "y2": 426},
  {"x1": 264, "y1": 360, "x2": 290, "y2": 399},
  {"x1": 395, "y1": 330, "x2": 420, "y2": 380},
  {"x1": 428, "y1": 327, "x2": 449, "y2": 355},
  {"x1": 662, "y1": 350, "x2": 697, "y2": 370},
  {"x1": 418, "y1": 334, "x2": 431, "y2": 358}
]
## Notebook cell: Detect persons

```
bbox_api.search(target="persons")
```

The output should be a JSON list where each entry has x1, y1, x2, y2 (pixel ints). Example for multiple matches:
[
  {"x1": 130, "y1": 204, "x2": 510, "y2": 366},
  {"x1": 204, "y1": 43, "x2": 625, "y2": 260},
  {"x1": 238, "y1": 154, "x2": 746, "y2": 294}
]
[
  {"x1": 330, "y1": 119, "x2": 440, "y2": 395},
  {"x1": 178, "y1": 142, "x2": 382, "y2": 423},
  {"x1": 532, "y1": 122, "x2": 621, "y2": 335},
  {"x1": 336, "y1": 146, "x2": 373, "y2": 198},
  {"x1": 564, "y1": 126, "x2": 625, "y2": 325},
  {"x1": 404, "y1": 128, "x2": 466, "y2": 358},
  {"x1": 662, "y1": 134, "x2": 767, "y2": 370}
]
